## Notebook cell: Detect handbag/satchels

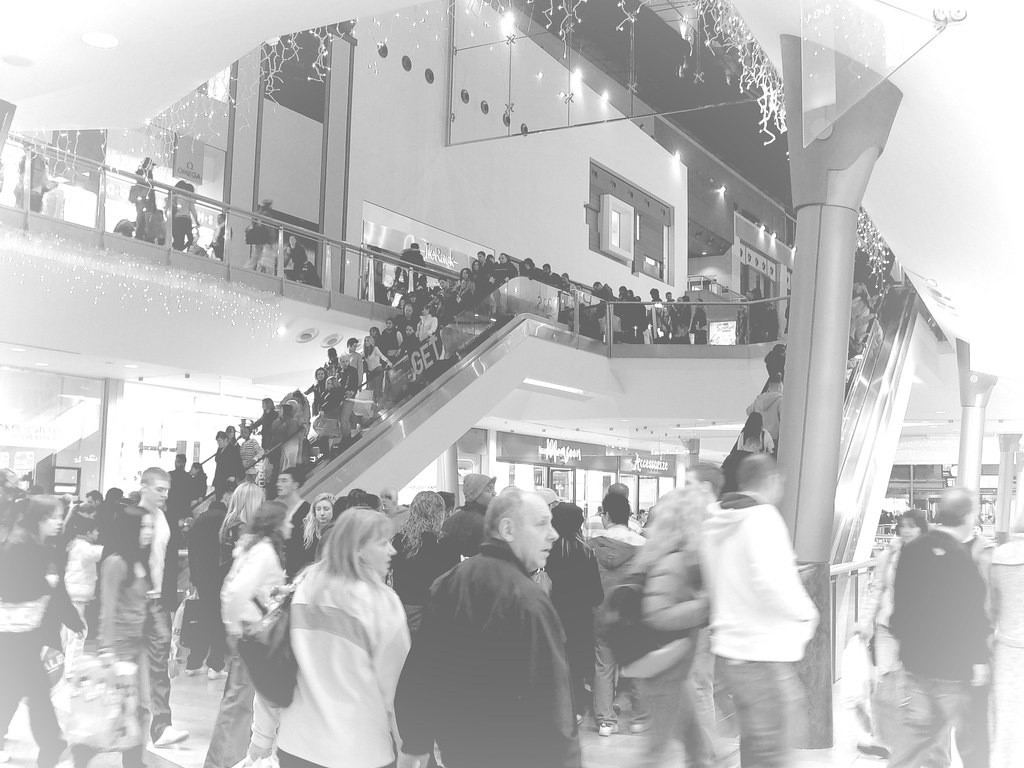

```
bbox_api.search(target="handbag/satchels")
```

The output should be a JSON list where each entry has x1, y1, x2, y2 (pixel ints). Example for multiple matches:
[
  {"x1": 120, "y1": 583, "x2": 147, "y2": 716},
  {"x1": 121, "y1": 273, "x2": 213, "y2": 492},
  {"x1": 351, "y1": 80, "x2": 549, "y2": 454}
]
[
  {"x1": 0, "y1": 536, "x2": 51, "y2": 633},
  {"x1": 52, "y1": 655, "x2": 143, "y2": 751},
  {"x1": 353, "y1": 390, "x2": 375, "y2": 416},
  {"x1": 236, "y1": 567, "x2": 297, "y2": 708}
]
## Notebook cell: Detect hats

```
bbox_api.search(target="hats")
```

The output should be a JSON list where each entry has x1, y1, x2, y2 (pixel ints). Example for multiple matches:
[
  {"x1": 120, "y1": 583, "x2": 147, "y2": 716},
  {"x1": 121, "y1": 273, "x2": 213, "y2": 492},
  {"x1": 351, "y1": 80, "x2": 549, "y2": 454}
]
[
  {"x1": 535, "y1": 485, "x2": 560, "y2": 507},
  {"x1": 463, "y1": 473, "x2": 497, "y2": 502}
]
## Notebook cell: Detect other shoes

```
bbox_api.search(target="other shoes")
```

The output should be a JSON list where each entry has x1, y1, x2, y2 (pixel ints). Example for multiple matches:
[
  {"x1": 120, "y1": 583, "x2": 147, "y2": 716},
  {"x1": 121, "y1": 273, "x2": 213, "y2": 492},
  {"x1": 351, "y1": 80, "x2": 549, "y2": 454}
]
[
  {"x1": 185, "y1": 664, "x2": 208, "y2": 677},
  {"x1": 210, "y1": 668, "x2": 229, "y2": 679},
  {"x1": 155, "y1": 724, "x2": 189, "y2": 748},
  {"x1": 600, "y1": 723, "x2": 617, "y2": 737},
  {"x1": 630, "y1": 720, "x2": 652, "y2": 735}
]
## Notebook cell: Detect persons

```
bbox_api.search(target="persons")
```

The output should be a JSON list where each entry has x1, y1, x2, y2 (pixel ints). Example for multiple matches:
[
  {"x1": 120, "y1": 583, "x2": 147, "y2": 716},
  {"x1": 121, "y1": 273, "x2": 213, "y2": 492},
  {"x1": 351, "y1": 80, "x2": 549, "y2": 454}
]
[{"x1": 0, "y1": 134, "x2": 996, "y2": 768}]
